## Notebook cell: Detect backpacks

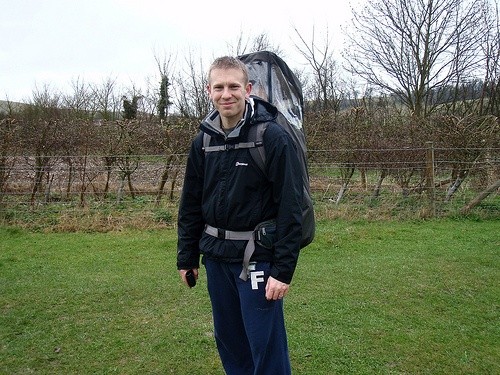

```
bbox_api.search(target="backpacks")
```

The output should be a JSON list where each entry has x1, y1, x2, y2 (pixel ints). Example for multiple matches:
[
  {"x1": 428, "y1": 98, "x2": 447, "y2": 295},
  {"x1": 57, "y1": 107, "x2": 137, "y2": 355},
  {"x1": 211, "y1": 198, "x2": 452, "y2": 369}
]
[{"x1": 202, "y1": 49, "x2": 311, "y2": 283}]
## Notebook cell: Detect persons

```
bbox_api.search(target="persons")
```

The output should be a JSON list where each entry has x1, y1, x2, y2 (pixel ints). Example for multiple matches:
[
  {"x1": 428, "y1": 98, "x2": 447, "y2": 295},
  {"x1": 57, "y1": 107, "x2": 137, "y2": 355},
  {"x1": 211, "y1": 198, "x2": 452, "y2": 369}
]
[{"x1": 175, "y1": 57, "x2": 316, "y2": 375}]
